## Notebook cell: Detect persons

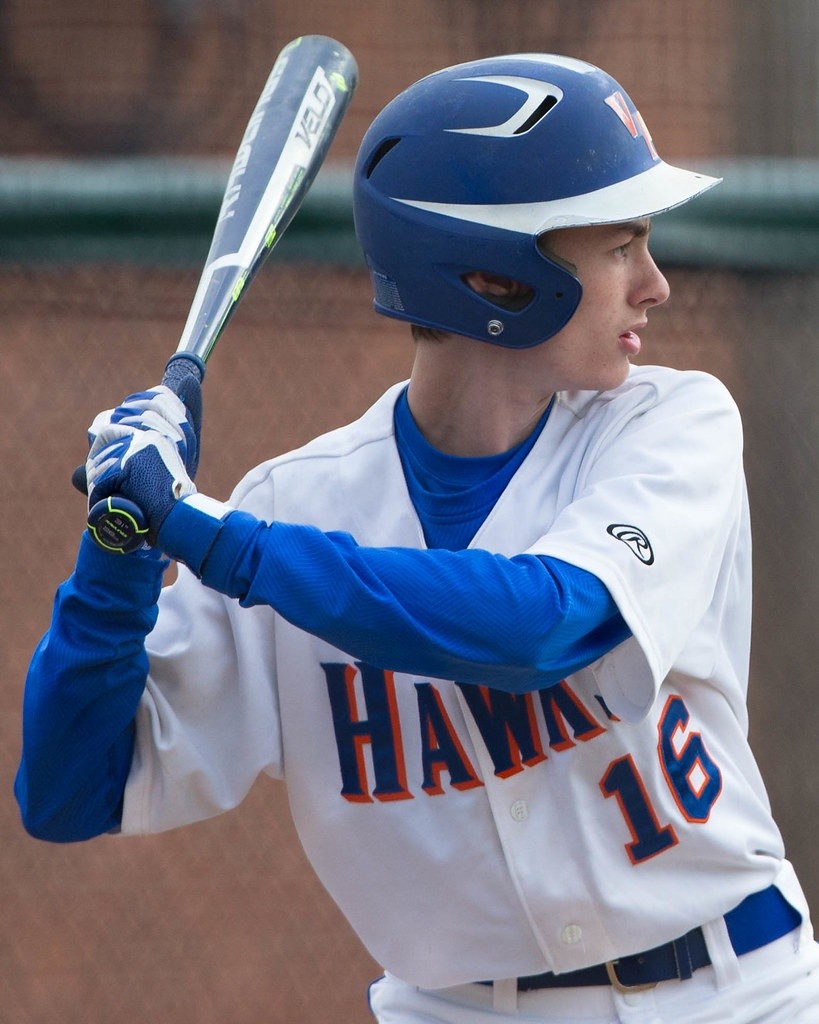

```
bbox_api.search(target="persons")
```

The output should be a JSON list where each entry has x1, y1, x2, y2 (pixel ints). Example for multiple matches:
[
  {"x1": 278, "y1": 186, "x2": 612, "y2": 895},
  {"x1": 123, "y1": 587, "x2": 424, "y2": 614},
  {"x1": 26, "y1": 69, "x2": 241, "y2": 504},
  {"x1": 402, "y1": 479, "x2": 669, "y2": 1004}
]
[{"x1": 13, "y1": 54, "x2": 819, "y2": 1024}]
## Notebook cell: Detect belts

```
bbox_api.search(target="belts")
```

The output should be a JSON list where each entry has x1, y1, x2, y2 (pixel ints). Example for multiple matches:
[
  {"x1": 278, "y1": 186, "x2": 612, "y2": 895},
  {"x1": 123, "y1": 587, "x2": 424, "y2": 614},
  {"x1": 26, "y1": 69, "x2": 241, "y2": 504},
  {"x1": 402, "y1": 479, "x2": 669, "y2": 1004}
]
[{"x1": 474, "y1": 885, "x2": 798, "y2": 992}]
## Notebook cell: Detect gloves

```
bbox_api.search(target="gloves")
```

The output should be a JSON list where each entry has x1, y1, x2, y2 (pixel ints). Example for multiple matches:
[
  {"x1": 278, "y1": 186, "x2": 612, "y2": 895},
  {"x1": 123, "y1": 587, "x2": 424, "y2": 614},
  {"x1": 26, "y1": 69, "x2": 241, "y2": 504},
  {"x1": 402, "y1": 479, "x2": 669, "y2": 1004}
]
[
  {"x1": 85, "y1": 422, "x2": 237, "y2": 580},
  {"x1": 71, "y1": 385, "x2": 199, "y2": 496}
]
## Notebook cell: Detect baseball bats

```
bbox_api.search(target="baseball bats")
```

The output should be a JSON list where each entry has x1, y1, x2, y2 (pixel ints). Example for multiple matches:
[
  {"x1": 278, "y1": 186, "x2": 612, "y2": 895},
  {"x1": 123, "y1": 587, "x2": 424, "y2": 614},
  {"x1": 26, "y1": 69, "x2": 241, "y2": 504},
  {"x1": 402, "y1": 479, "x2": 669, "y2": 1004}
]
[{"x1": 85, "y1": 33, "x2": 359, "y2": 553}]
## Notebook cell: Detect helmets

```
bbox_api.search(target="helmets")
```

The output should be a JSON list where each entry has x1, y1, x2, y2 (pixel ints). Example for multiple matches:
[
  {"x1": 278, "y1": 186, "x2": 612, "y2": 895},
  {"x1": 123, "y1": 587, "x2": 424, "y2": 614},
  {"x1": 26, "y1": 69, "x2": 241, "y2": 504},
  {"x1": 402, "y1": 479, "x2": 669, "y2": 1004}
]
[{"x1": 353, "y1": 53, "x2": 723, "y2": 351}]
